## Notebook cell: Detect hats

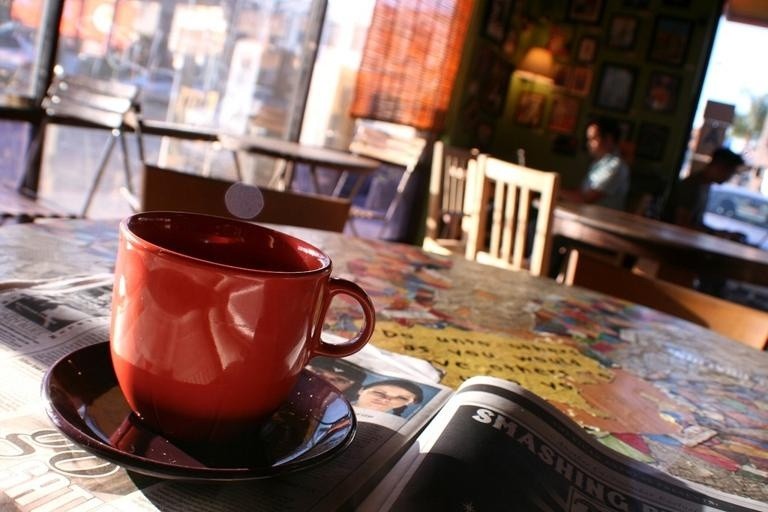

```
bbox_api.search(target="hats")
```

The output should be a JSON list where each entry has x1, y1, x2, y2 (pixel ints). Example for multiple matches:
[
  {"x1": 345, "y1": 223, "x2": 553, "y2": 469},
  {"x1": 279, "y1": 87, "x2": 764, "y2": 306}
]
[{"x1": 309, "y1": 355, "x2": 367, "y2": 385}]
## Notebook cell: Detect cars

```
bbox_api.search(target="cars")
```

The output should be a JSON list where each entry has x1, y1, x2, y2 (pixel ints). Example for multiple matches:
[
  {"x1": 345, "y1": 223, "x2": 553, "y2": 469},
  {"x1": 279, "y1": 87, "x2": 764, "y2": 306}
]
[
  {"x1": 0, "y1": 20, "x2": 80, "y2": 98},
  {"x1": 702, "y1": 182, "x2": 768, "y2": 252},
  {"x1": 124, "y1": 63, "x2": 181, "y2": 102}
]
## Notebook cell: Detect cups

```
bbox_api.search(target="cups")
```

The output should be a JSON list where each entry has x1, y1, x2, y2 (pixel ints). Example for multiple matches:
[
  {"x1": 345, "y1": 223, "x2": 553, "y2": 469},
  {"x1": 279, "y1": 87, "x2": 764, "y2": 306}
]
[{"x1": 111, "y1": 208, "x2": 376, "y2": 448}]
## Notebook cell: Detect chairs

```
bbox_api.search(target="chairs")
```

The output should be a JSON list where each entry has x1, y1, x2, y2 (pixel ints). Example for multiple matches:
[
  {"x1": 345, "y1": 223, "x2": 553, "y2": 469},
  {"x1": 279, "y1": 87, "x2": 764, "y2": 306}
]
[
  {"x1": 142, "y1": 165, "x2": 352, "y2": 234},
  {"x1": 564, "y1": 248, "x2": 768, "y2": 351},
  {"x1": 458, "y1": 156, "x2": 559, "y2": 276},
  {"x1": 517, "y1": 150, "x2": 529, "y2": 168},
  {"x1": 0, "y1": 71, "x2": 139, "y2": 216},
  {"x1": 329, "y1": 117, "x2": 427, "y2": 239},
  {"x1": 432, "y1": 142, "x2": 482, "y2": 254}
]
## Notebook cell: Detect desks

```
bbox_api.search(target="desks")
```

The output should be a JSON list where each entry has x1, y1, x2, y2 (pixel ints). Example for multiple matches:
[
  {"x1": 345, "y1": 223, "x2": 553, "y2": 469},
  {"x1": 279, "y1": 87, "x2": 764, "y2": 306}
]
[
  {"x1": 532, "y1": 197, "x2": 768, "y2": 289},
  {"x1": 237, "y1": 137, "x2": 380, "y2": 208},
  {"x1": 0, "y1": 217, "x2": 768, "y2": 512},
  {"x1": 0, "y1": 104, "x2": 219, "y2": 223}
]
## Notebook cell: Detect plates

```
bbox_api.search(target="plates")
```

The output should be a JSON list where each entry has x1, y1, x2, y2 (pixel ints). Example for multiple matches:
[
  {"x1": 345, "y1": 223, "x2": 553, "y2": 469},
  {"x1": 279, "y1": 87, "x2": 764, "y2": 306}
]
[{"x1": 41, "y1": 337, "x2": 358, "y2": 485}]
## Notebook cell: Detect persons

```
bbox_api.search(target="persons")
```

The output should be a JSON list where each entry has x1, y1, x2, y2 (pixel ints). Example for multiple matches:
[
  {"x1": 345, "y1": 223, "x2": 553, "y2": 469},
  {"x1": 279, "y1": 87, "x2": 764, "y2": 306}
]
[
  {"x1": 526, "y1": 118, "x2": 632, "y2": 277},
  {"x1": 305, "y1": 354, "x2": 365, "y2": 400},
  {"x1": 661, "y1": 147, "x2": 749, "y2": 296},
  {"x1": 351, "y1": 380, "x2": 422, "y2": 417}
]
